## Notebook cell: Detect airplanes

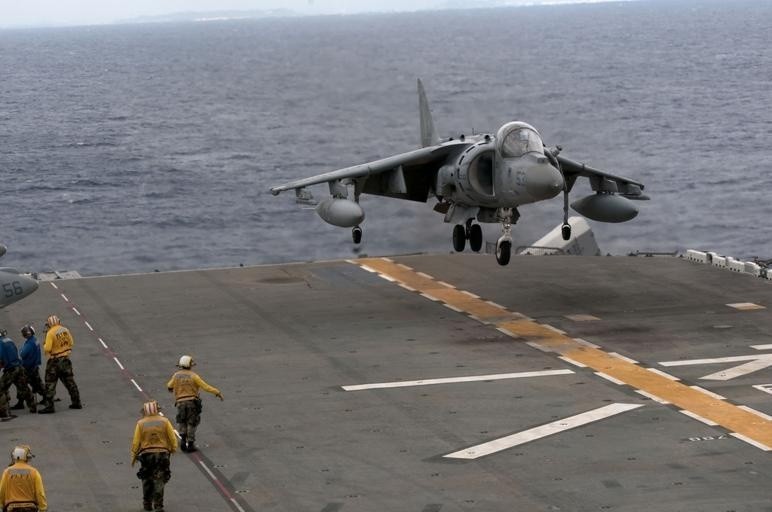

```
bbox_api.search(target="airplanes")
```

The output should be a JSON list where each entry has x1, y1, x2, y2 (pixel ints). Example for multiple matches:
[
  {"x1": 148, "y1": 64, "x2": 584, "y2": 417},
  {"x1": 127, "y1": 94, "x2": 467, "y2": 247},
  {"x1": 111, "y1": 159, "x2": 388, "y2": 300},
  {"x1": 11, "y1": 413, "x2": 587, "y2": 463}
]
[
  {"x1": 267, "y1": 76, "x2": 651, "y2": 266},
  {"x1": 0, "y1": 240, "x2": 40, "y2": 308}
]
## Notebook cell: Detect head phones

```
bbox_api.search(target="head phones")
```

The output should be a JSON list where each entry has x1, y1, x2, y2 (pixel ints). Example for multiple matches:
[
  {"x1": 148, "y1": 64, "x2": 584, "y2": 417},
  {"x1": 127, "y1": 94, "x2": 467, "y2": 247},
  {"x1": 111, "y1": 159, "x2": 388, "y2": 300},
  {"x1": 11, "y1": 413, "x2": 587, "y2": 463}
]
[
  {"x1": 45, "y1": 319, "x2": 61, "y2": 326},
  {"x1": 141, "y1": 399, "x2": 161, "y2": 414},
  {"x1": 11, "y1": 446, "x2": 33, "y2": 460},
  {"x1": 176, "y1": 360, "x2": 196, "y2": 369},
  {"x1": 25, "y1": 325, "x2": 32, "y2": 337}
]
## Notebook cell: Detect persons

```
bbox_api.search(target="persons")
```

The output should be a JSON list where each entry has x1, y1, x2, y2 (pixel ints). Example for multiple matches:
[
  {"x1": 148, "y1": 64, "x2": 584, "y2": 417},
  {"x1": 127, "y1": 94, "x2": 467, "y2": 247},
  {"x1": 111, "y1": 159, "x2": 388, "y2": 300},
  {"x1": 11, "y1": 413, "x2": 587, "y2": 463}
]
[
  {"x1": 166, "y1": 355, "x2": 225, "y2": 453},
  {"x1": 130, "y1": 398, "x2": 178, "y2": 511},
  {"x1": 0, "y1": 314, "x2": 82, "y2": 421},
  {"x1": 0, "y1": 443, "x2": 49, "y2": 511}
]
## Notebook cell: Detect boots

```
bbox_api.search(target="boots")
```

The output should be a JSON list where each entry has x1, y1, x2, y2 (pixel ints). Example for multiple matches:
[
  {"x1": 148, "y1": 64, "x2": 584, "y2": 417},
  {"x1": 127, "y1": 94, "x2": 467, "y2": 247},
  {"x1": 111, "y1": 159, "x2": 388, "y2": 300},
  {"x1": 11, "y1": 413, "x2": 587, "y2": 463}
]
[
  {"x1": 180, "y1": 442, "x2": 197, "y2": 453},
  {"x1": 10, "y1": 400, "x2": 24, "y2": 409},
  {"x1": 69, "y1": 400, "x2": 82, "y2": 409},
  {"x1": 25, "y1": 402, "x2": 57, "y2": 414}
]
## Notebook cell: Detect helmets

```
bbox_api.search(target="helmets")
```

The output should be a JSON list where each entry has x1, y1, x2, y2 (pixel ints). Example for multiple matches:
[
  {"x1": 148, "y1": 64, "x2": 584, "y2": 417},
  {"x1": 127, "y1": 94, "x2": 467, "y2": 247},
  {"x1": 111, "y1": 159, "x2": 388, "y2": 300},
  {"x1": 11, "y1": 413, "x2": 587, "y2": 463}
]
[
  {"x1": 20, "y1": 325, "x2": 36, "y2": 337},
  {"x1": 46, "y1": 315, "x2": 61, "y2": 328},
  {"x1": 179, "y1": 355, "x2": 195, "y2": 369},
  {"x1": 10, "y1": 443, "x2": 34, "y2": 462},
  {"x1": 143, "y1": 399, "x2": 160, "y2": 416},
  {"x1": 1, "y1": 327, "x2": 9, "y2": 336}
]
